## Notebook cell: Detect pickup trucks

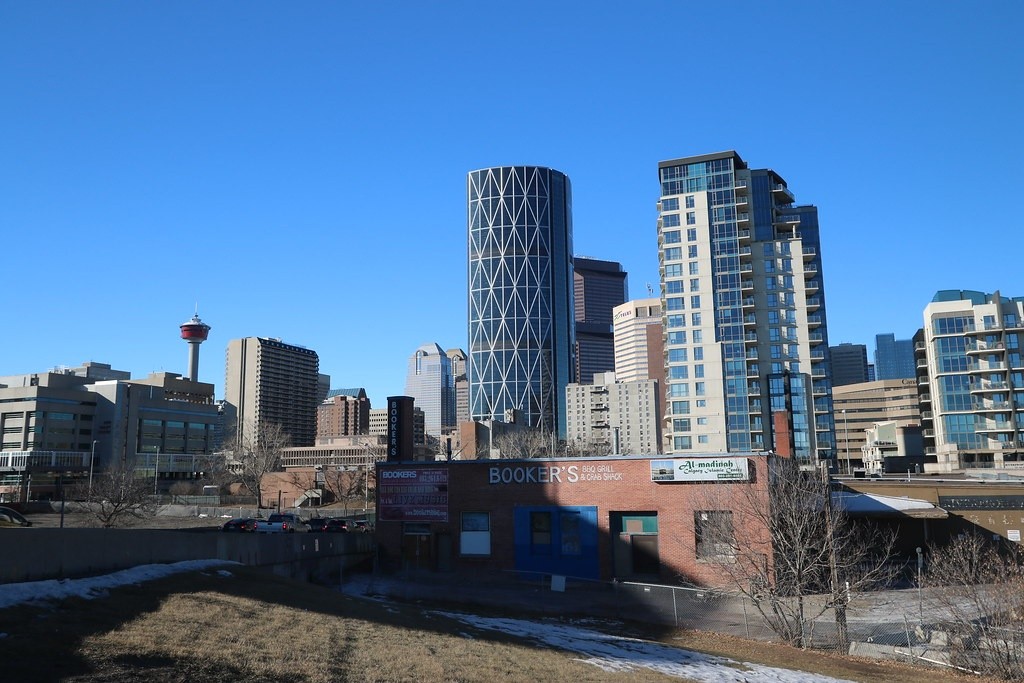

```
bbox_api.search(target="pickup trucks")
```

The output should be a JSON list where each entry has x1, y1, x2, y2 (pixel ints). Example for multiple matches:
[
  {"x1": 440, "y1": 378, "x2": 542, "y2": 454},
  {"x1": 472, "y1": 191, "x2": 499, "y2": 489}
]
[{"x1": 253, "y1": 512, "x2": 308, "y2": 535}]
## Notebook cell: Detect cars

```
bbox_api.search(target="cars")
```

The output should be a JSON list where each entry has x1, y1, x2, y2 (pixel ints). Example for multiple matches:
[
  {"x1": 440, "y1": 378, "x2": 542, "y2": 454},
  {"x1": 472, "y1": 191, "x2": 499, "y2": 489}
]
[
  {"x1": 216, "y1": 518, "x2": 258, "y2": 535},
  {"x1": 326, "y1": 518, "x2": 356, "y2": 534},
  {"x1": 352, "y1": 520, "x2": 372, "y2": 534},
  {"x1": 0, "y1": 506, "x2": 33, "y2": 527},
  {"x1": 305, "y1": 517, "x2": 328, "y2": 532}
]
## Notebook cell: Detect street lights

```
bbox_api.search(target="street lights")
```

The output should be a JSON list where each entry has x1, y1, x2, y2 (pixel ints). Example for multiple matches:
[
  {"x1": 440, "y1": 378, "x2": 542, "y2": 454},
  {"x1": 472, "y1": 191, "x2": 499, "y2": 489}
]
[
  {"x1": 153, "y1": 445, "x2": 160, "y2": 494},
  {"x1": 364, "y1": 443, "x2": 368, "y2": 509},
  {"x1": 88, "y1": 440, "x2": 100, "y2": 502},
  {"x1": 842, "y1": 409, "x2": 851, "y2": 474}
]
